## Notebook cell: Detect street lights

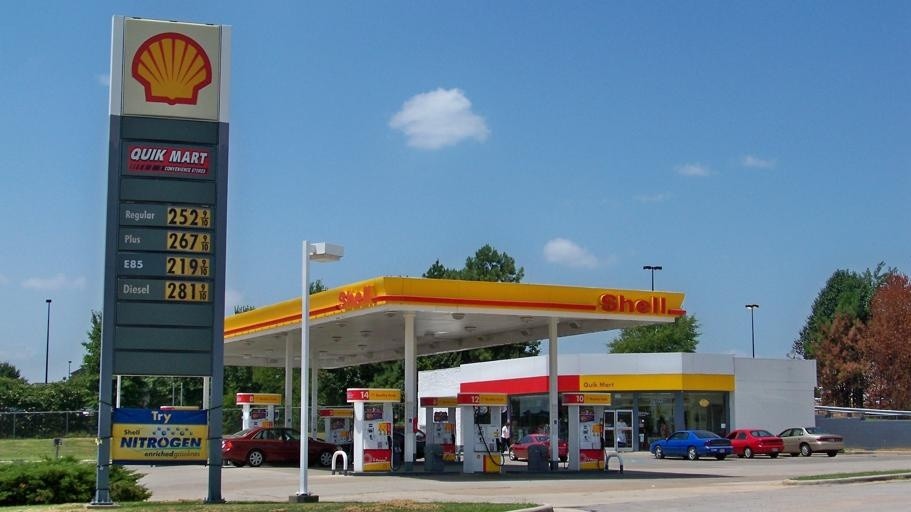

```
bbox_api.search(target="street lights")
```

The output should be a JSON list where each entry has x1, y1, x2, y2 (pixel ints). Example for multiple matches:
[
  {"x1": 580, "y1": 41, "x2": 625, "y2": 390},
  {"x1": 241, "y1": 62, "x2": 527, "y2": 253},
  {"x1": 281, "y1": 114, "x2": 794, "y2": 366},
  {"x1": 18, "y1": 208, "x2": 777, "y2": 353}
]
[
  {"x1": 643, "y1": 266, "x2": 662, "y2": 291},
  {"x1": 745, "y1": 304, "x2": 759, "y2": 358},
  {"x1": 45, "y1": 300, "x2": 51, "y2": 385},
  {"x1": 62, "y1": 361, "x2": 71, "y2": 383},
  {"x1": 286, "y1": 240, "x2": 345, "y2": 502}
]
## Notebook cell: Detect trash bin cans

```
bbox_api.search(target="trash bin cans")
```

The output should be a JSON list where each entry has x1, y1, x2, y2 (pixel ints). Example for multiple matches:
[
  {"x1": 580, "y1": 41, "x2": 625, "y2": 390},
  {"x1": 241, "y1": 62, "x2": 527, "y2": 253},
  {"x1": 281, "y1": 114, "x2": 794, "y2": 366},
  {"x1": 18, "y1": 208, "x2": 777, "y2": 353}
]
[{"x1": 528, "y1": 444, "x2": 549, "y2": 474}]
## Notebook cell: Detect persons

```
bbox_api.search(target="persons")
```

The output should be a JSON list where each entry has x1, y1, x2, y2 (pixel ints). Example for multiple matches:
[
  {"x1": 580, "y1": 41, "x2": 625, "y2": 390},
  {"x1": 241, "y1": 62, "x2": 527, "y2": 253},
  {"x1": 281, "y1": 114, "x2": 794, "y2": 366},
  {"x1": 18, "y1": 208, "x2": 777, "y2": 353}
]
[
  {"x1": 501, "y1": 423, "x2": 510, "y2": 453},
  {"x1": 615, "y1": 418, "x2": 628, "y2": 446}
]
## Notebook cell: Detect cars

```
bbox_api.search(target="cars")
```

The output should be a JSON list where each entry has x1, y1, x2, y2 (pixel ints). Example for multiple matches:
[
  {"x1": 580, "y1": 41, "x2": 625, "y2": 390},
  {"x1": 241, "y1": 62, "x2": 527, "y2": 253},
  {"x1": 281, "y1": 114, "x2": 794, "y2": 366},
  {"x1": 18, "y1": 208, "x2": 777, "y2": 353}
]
[
  {"x1": 338, "y1": 426, "x2": 427, "y2": 465},
  {"x1": 222, "y1": 427, "x2": 343, "y2": 467},
  {"x1": 650, "y1": 427, "x2": 843, "y2": 460},
  {"x1": 508, "y1": 433, "x2": 568, "y2": 462}
]
